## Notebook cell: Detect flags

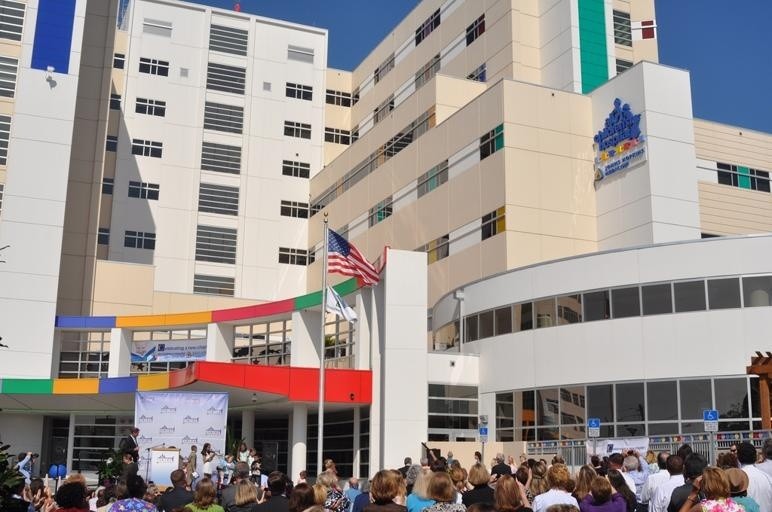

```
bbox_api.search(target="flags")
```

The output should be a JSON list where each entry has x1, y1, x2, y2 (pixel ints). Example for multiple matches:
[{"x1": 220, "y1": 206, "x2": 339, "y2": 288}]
[
  {"x1": 326, "y1": 284, "x2": 356, "y2": 325},
  {"x1": 328, "y1": 227, "x2": 381, "y2": 290}
]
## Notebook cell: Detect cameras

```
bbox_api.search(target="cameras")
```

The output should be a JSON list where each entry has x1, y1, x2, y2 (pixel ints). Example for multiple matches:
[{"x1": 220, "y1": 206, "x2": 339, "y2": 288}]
[
  {"x1": 30, "y1": 453, "x2": 39, "y2": 461},
  {"x1": 217, "y1": 465, "x2": 226, "y2": 472}
]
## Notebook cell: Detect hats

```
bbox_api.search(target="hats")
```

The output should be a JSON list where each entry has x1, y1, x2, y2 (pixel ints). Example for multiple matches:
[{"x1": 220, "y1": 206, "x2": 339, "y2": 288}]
[{"x1": 724, "y1": 467, "x2": 749, "y2": 493}]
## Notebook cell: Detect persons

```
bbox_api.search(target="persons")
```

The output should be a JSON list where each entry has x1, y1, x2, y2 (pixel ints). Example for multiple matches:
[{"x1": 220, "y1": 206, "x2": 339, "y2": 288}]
[{"x1": 12, "y1": 427, "x2": 771, "y2": 512}]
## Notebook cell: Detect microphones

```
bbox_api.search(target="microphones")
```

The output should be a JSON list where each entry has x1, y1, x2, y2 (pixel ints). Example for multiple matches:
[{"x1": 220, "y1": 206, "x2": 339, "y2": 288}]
[{"x1": 163, "y1": 443, "x2": 165, "y2": 446}]
